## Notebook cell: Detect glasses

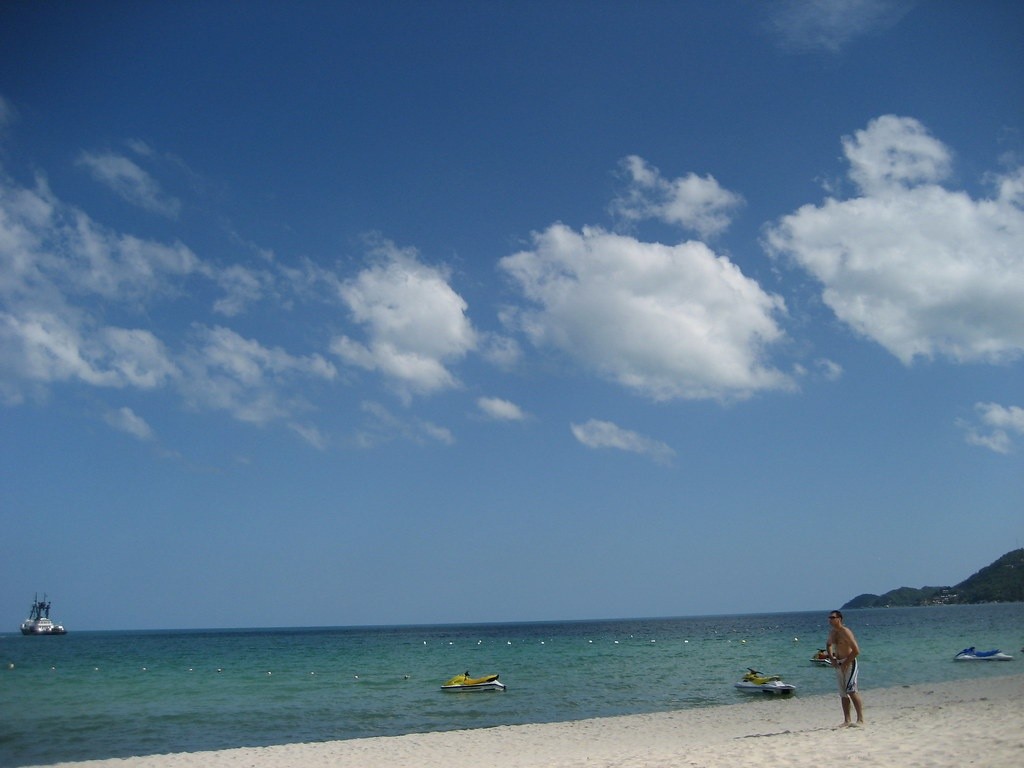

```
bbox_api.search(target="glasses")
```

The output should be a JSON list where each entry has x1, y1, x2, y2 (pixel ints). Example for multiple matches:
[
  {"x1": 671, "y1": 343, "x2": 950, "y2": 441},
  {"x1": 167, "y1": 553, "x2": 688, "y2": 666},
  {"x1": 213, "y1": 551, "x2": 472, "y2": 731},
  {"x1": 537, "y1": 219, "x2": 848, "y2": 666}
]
[{"x1": 828, "y1": 616, "x2": 839, "y2": 619}]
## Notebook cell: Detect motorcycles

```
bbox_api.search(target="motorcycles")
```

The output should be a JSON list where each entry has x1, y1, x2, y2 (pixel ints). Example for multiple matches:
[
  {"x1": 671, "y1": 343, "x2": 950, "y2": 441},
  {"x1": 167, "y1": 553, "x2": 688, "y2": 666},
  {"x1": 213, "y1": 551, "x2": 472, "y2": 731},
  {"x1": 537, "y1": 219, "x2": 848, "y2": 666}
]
[
  {"x1": 734, "y1": 667, "x2": 797, "y2": 696},
  {"x1": 810, "y1": 647, "x2": 836, "y2": 667},
  {"x1": 440, "y1": 670, "x2": 507, "y2": 692},
  {"x1": 954, "y1": 646, "x2": 1016, "y2": 662}
]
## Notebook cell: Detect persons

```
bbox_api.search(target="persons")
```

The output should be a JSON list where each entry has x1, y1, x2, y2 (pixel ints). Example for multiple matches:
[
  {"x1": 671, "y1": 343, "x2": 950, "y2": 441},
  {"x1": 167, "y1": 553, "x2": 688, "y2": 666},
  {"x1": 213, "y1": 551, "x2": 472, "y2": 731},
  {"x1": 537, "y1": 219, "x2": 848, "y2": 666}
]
[{"x1": 825, "y1": 610, "x2": 863, "y2": 727}]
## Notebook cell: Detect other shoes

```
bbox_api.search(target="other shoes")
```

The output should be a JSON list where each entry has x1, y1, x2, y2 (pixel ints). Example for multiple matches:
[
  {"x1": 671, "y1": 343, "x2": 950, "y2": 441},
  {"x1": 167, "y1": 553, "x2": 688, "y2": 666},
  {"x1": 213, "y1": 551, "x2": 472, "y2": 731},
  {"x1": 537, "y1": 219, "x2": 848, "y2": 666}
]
[{"x1": 849, "y1": 722, "x2": 864, "y2": 727}]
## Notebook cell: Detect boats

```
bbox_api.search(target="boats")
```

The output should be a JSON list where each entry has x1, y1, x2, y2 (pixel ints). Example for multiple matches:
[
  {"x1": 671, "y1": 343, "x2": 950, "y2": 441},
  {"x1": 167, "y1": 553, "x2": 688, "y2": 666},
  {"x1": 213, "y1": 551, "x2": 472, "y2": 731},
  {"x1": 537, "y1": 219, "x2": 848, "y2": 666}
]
[{"x1": 20, "y1": 592, "x2": 68, "y2": 636}]
